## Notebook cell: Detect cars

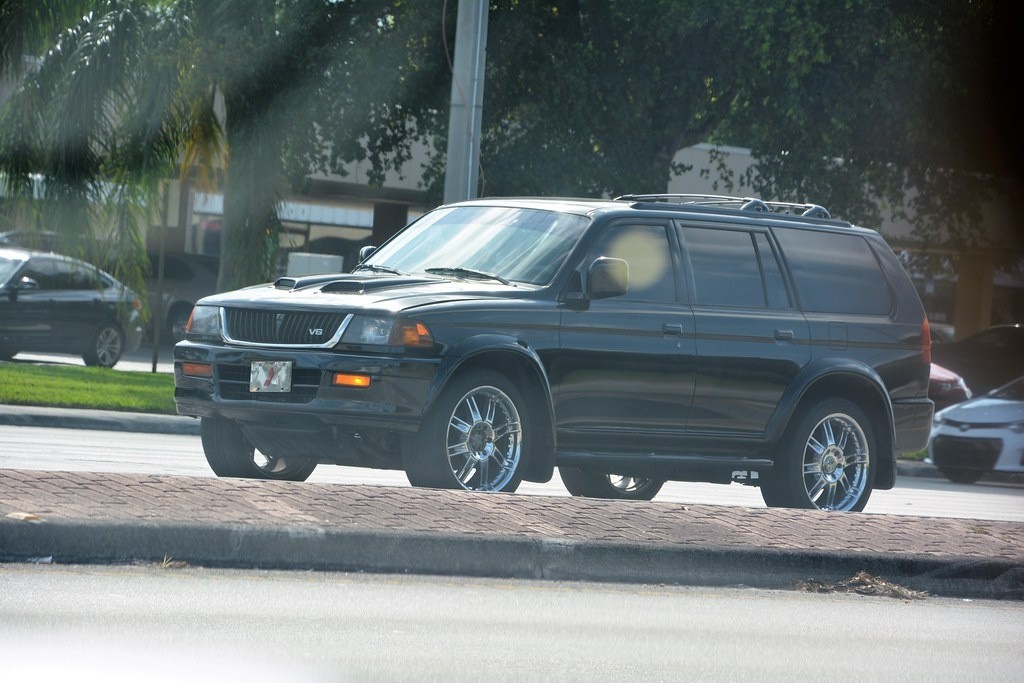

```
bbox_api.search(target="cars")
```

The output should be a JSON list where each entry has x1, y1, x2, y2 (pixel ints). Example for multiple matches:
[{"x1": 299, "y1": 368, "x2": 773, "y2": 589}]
[
  {"x1": 0, "y1": 230, "x2": 220, "y2": 346},
  {"x1": 928, "y1": 317, "x2": 1023, "y2": 484},
  {"x1": 0, "y1": 245, "x2": 148, "y2": 370}
]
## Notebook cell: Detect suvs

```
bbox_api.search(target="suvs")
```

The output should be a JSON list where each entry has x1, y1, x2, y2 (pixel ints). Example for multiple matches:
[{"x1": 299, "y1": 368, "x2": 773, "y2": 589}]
[{"x1": 175, "y1": 190, "x2": 934, "y2": 515}]
